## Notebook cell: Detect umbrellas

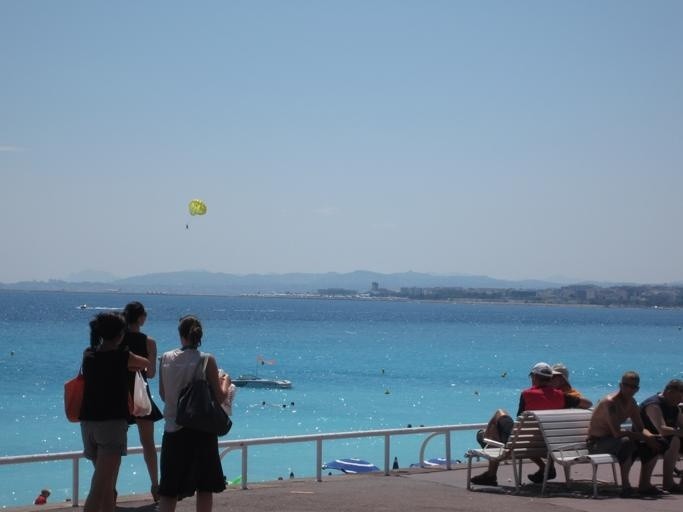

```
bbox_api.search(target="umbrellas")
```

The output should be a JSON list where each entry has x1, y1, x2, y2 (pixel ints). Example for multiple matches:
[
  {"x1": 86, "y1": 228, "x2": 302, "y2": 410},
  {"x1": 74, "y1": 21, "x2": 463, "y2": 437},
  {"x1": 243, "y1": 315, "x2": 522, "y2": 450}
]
[
  {"x1": 324, "y1": 456, "x2": 378, "y2": 473},
  {"x1": 392, "y1": 455, "x2": 399, "y2": 468},
  {"x1": 408, "y1": 457, "x2": 457, "y2": 469}
]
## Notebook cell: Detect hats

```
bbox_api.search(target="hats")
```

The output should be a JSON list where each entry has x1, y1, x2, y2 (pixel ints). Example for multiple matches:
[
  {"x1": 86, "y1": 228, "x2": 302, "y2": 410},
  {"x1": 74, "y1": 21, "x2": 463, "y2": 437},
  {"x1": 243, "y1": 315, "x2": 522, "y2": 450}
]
[
  {"x1": 552, "y1": 363, "x2": 572, "y2": 389},
  {"x1": 528, "y1": 362, "x2": 553, "y2": 378}
]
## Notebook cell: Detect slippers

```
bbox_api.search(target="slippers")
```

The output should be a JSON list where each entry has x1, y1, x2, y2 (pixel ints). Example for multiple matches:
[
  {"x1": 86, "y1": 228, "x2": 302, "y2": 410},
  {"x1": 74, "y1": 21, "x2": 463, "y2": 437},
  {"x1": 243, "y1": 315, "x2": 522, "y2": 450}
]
[
  {"x1": 640, "y1": 486, "x2": 664, "y2": 499},
  {"x1": 620, "y1": 486, "x2": 642, "y2": 499},
  {"x1": 666, "y1": 482, "x2": 682, "y2": 495}
]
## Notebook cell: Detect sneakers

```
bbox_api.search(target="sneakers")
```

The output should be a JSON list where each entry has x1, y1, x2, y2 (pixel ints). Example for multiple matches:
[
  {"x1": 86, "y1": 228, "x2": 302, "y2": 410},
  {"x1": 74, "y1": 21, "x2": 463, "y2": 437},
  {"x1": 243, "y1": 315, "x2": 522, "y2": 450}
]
[
  {"x1": 528, "y1": 467, "x2": 555, "y2": 483},
  {"x1": 471, "y1": 470, "x2": 498, "y2": 486},
  {"x1": 477, "y1": 429, "x2": 487, "y2": 449}
]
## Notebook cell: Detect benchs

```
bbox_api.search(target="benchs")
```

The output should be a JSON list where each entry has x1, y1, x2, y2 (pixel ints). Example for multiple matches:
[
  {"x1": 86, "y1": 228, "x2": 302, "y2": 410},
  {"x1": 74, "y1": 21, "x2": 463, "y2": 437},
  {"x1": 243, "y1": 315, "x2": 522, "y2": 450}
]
[{"x1": 466, "y1": 408, "x2": 622, "y2": 496}]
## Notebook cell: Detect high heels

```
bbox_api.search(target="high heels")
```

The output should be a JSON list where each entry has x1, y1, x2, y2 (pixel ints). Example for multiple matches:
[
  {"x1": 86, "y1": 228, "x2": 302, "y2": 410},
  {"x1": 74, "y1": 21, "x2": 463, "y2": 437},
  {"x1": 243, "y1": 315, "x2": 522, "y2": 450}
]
[{"x1": 151, "y1": 484, "x2": 161, "y2": 504}]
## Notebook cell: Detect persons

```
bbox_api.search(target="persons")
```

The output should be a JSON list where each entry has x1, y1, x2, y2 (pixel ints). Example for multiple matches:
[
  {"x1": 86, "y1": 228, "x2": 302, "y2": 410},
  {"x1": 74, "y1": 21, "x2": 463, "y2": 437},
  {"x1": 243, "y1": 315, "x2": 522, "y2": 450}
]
[
  {"x1": 78, "y1": 310, "x2": 151, "y2": 512},
  {"x1": 585, "y1": 370, "x2": 665, "y2": 501},
  {"x1": 630, "y1": 377, "x2": 682, "y2": 496},
  {"x1": 528, "y1": 363, "x2": 581, "y2": 483},
  {"x1": 154, "y1": 314, "x2": 231, "y2": 511},
  {"x1": 119, "y1": 301, "x2": 160, "y2": 501},
  {"x1": 469, "y1": 362, "x2": 595, "y2": 486},
  {"x1": 32, "y1": 489, "x2": 50, "y2": 505}
]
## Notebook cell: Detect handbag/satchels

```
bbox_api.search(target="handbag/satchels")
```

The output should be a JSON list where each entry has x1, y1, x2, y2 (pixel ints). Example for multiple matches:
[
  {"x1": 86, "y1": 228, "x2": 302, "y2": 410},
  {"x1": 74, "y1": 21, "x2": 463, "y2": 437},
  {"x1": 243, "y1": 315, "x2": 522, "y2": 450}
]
[
  {"x1": 64, "y1": 364, "x2": 86, "y2": 422},
  {"x1": 174, "y1": 352, "x2": 232, "y2": 437}
]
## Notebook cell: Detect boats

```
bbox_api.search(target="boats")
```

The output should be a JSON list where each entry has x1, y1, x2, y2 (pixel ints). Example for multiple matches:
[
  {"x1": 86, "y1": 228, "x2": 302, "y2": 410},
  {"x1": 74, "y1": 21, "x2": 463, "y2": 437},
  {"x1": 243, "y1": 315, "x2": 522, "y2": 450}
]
[{"x1": 231, "y1": 374, "x2": 290, "y2": 389}]
